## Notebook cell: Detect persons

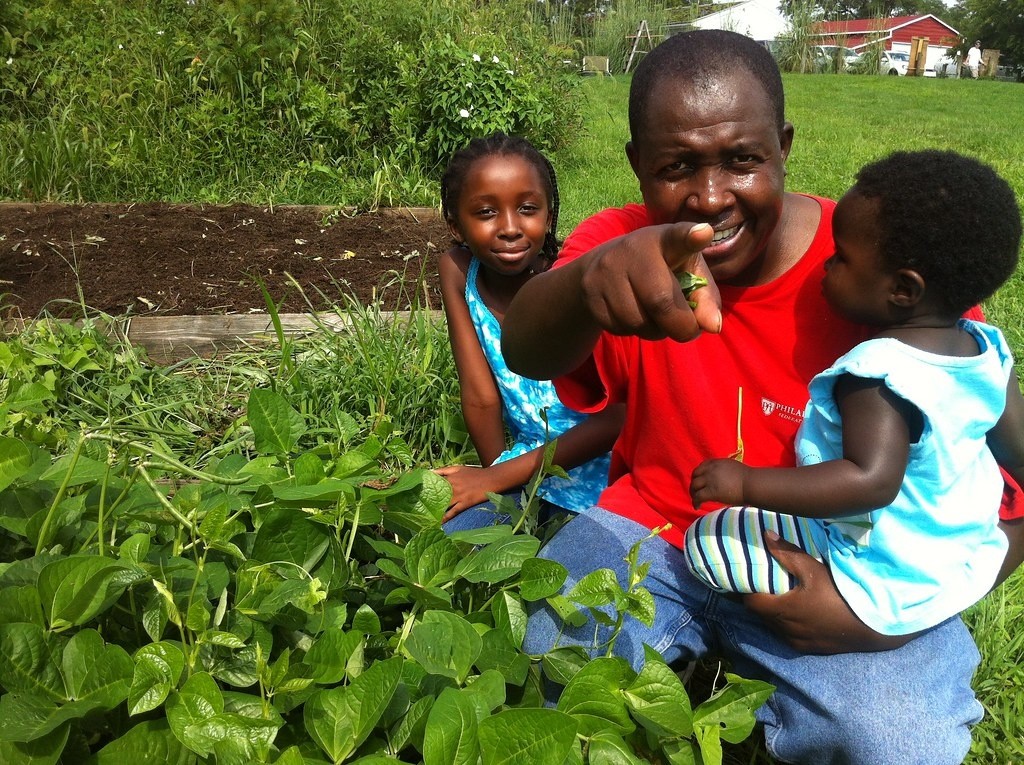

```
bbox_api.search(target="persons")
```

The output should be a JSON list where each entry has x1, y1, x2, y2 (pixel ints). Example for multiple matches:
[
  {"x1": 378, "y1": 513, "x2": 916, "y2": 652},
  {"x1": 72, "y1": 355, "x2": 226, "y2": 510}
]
[
  {"x1": 430, "y1": 132, "x2": 614, "y2": 551},
  {"x1": 683, "y1": 148, "x2": 1024, "y2": 636},
  {"x1": 500, "y1": 29, "x2": 1024, "y2": 765}
]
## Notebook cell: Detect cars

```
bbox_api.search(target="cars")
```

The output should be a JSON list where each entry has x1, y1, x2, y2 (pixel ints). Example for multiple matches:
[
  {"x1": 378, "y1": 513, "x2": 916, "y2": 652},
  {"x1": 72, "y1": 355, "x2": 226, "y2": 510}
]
[
  {"x1": 808, "y1": 44, "x2": 867, "y2": 74},
  {"x1": 861, "y1": 50, "x2": 910, "y2": 77}
]
